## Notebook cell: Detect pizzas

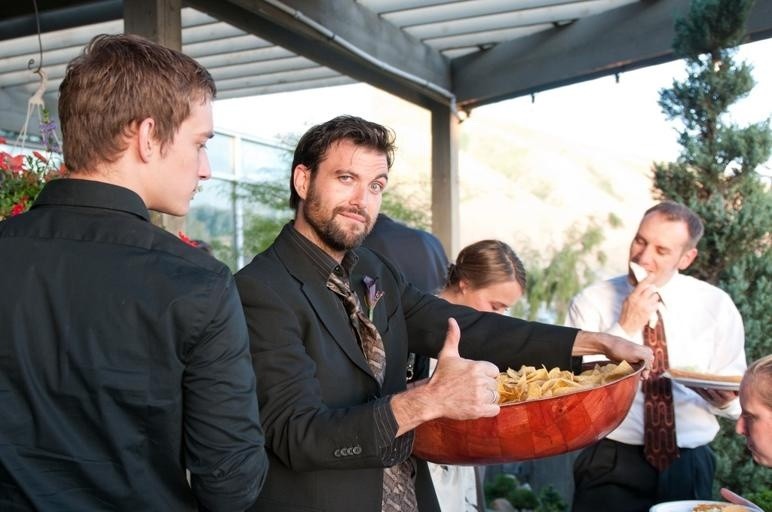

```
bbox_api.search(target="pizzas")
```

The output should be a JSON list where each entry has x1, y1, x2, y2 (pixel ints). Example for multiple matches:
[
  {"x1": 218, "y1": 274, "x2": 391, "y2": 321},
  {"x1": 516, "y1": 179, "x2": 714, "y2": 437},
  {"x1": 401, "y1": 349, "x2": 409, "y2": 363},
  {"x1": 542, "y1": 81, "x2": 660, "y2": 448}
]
[
  {"x1": 668, "y1": 368, "x2": 743, "y2": 383},
  {"x1": 691, "y1": 504, "x2": 751, "y2": 512}
]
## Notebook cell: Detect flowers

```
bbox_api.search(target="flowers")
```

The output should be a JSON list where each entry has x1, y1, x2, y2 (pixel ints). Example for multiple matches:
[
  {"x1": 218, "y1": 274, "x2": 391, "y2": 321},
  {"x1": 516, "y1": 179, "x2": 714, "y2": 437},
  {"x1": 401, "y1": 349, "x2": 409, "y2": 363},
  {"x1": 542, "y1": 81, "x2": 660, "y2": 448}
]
[{"x1": 0, "y1": 108, "x2": 201, "y2": 252}]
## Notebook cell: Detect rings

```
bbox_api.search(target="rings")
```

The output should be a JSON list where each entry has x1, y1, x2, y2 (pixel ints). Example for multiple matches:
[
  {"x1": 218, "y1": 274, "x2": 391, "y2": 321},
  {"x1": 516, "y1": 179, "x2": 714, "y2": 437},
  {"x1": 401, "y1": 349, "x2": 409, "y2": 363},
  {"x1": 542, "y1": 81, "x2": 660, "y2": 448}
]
[{"x1": 487, "y1": 384, "x2": 496, "y2": 405}]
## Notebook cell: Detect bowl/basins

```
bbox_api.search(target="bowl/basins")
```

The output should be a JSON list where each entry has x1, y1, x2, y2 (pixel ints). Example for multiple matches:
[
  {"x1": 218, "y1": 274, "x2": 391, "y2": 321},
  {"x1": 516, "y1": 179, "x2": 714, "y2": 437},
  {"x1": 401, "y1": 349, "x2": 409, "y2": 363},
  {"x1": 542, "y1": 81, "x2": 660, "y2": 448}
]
[{"x1": 407, "y1": 360, "x2": 652, "y2": 465}]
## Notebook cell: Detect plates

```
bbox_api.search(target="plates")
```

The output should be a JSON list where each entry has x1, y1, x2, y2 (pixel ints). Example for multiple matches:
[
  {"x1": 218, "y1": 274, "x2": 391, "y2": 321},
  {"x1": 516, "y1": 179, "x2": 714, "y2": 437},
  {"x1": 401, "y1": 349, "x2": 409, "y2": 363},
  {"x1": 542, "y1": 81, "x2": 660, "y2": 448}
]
[
  {"x1": 672, "y1": 378, "x2": 740, "y2": 391},
  {"x1": 648, "y1": 499, "x2": 762, "y2": 512}
]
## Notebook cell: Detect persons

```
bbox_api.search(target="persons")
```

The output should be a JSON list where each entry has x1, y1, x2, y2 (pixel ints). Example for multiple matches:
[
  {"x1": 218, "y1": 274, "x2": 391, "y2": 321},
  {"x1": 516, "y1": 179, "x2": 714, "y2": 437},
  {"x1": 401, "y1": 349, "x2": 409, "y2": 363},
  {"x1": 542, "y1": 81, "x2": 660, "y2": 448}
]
[
  {"x1": 0, "y1": 30, "x2": 272, "y2": 510},
  {"x1": 406, "y1": 239, "x2": 527, "y2": 512},
  {"x1": 368, "y1": 214, "x2": 450, "y2": 295},
  {"x1": 232, "y1": 112, "x2": 656, "y2": 512},
  {"x1": 718, "y1": 351, "x2": 772, "y2": 512},
  {"x1": 566, "y1": 200, "x2": 750, "y2": 512}
]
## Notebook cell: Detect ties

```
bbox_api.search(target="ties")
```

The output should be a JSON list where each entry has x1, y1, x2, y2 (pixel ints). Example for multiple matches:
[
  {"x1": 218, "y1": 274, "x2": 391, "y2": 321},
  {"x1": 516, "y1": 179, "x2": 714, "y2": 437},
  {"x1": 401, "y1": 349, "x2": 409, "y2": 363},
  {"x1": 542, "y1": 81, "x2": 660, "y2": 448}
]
[
  {"x1": 325, "y1": 273, "x2": 420, "y2": 512},
  {"x1": 640, "y1": 292, "x2": 678, "y2": 470}
]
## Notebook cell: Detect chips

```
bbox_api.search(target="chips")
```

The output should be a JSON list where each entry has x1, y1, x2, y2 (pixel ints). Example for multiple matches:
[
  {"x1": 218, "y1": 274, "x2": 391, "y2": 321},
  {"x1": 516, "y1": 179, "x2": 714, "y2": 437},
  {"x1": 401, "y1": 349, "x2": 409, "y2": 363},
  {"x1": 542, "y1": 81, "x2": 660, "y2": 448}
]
[
  {"x1": 630, "y1": 261, "x2": 647, "y2": 284},
  {"x1": 495, "y1": 360, "x2": 636, "y2": 405}
]
